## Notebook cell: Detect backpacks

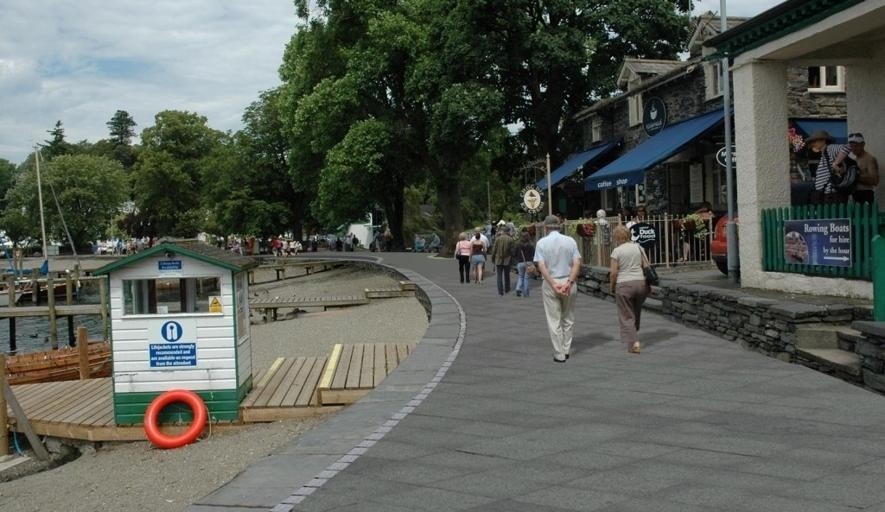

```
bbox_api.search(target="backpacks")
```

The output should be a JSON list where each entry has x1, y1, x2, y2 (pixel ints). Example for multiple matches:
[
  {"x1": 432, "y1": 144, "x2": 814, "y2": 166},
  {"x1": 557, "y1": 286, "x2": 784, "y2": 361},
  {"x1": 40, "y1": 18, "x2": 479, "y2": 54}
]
[{"x1": 824, "y1": 146, "x2": 857, "y2": 191}]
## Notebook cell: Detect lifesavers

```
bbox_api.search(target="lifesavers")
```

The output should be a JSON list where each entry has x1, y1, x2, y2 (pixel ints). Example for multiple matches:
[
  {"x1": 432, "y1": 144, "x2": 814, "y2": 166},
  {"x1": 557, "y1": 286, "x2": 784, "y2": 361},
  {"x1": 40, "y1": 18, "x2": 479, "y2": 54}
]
[{"x1": 144, "y1": 391, "x2": 207, "y2": 448}]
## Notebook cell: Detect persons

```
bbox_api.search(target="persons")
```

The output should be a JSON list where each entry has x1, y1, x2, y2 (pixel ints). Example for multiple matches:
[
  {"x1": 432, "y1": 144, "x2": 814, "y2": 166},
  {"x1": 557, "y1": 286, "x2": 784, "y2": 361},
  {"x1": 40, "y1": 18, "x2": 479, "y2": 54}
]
[
  {"x1": 807, "y1": 130, "x2": 852, "y2": 203},
  {"x1": 454, "y1": 219, "x2": 535, "y2": 297},
  {"x1": 848, "y1": 133, "x2": 878, "y2": 203},
  {"x1": 622, "y1": 206, "x2": 634, "y2": 221},
  {"x1": 116, "y1": 239, "x2": 138, "y2": 255},
  {"x1": 533, "y1": 215, "x2": 582, "y2": 362},
  {"x1": 677, "y1": 201, "x2": 715, "y2": 262},
  {"x1": 632, "y1": 206, "x2": 647, "y2": 220},
  {"x1": 271, "y1": 233, "x2": 440, "y2": 256},
  {"x1": 609, "y1": 225, "x2": 650, "y2": 353}
]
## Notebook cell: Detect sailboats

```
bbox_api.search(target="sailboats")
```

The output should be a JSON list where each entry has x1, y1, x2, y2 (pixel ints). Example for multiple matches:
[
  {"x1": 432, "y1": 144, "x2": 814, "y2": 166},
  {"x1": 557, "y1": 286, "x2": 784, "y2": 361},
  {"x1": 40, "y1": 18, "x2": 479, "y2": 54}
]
[{"x1": 0, "y1": 144, "x2": 88, "y2": 306}]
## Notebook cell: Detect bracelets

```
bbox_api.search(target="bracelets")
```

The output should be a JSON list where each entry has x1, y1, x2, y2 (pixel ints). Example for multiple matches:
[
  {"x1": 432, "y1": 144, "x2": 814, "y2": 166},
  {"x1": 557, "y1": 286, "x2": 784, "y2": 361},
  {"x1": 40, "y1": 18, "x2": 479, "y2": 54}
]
[{"x1": 567, "y1": 280, "x2": 574, "y2": 284}]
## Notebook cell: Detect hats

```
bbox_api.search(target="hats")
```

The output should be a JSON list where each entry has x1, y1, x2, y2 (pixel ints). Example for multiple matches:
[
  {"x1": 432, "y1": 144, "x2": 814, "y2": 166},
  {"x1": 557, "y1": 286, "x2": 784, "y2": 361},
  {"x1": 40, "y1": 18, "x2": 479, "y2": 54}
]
[
  {"x1": 848, "y1": 135, "x2": 864, "y2": 144},
  {"x1": 806, "y1": 131, "x2": 836, "y2": 141}
]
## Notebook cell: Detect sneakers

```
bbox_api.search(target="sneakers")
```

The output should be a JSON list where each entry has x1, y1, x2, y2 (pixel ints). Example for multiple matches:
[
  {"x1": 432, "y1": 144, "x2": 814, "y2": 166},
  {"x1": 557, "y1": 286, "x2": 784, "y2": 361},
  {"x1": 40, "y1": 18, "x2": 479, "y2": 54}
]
[
  {"x1": 499, "y1": 288, "x2": 522, "y2": 296},
  {"x1": 554, "y1": 357, "x2": 565, "y2": 362},
  {"x1": 566, "y1": 355, "x2": 570, "y2": 359},
  {"x1": 630, "y1": 341, "x2": 640, "y2": 354}
]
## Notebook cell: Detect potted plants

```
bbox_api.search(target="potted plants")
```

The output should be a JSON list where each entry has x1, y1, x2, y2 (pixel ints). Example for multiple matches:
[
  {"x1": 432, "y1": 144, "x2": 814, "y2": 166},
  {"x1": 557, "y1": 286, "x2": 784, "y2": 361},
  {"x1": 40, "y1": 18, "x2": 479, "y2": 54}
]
[
  {"x1": 683, "y1": 213, "x2": 708, "y2": 238},
  {"x1": 567, "y1": 217, "x2": 597, "y2": 238}
]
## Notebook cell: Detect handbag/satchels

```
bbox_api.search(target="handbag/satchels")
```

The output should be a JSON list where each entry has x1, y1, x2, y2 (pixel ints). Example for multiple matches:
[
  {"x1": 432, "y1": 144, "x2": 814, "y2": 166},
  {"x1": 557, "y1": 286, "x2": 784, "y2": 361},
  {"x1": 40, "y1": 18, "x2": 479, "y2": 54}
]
[
  {"x1": 456, "y1": 251, "x2": 461, "y2": 259},
  {"x1": 527, "y1": 264, "x2": 536, "y2": 274},
  {"x1": 644, "y1": 265, "x2": 658, "y2": 286}
]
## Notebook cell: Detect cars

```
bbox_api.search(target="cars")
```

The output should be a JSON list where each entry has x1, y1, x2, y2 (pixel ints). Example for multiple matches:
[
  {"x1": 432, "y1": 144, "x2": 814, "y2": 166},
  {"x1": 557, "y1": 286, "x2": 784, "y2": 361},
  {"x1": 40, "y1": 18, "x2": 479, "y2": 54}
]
[{"x1": 710, "y1": 209, "x2": 741, "y2": 278}]
